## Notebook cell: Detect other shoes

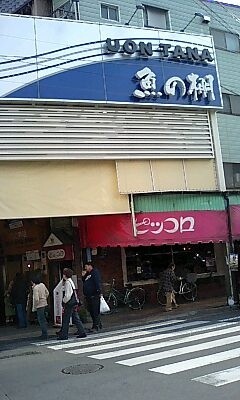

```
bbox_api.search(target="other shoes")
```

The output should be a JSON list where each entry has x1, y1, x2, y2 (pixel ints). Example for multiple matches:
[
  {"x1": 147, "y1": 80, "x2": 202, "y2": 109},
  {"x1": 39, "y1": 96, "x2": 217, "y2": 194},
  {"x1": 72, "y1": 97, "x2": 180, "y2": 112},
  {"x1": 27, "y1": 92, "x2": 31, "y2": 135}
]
[
  {"x1": 74, "y1": 331, "x2": 85, "y2": 335},
  {"x1": 38, "y1": 335, "x2": 48, "y2": 341},
  {"x1": 76, "y1": 334, "x2": 86, "y2": 338},
  {"x1": 165, "y1": 308, "x2": 172, "y2": 312},
  {"x1": 88, "y1": 327, "x2": 98, "y2": 331},
  {"x1": 56, "y1": 332, "x2": 61, "y2": 335},
  {"x1": 174, "y1": 305, "x2": 180, "y2": 309},
  {"x1": 57, "y1": 336, "x2": 68, "y2": 340}
]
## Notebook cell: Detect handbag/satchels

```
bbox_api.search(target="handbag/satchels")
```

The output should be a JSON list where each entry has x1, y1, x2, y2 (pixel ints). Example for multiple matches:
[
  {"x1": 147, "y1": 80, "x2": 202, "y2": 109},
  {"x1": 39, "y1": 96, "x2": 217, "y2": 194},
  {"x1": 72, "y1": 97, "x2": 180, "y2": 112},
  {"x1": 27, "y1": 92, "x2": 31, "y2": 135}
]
[
  {"x1": 100, "y1": 293, "x2": 110, "y2": 313},
  {"x1": 62, "y1": 280, "x2": 78, "y2": 309}
]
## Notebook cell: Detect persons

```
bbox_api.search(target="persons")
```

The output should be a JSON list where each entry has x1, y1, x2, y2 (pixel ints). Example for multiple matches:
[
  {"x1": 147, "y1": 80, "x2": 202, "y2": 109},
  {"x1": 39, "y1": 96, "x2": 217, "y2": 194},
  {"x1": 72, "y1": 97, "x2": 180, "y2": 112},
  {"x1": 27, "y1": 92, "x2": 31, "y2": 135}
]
[
  {"x1": 56, "y1": 269, "x2": 88, "y2": 339},
  {"x1": 81, "y1": 269, "x2": 87, "y2": 278},
  {"x1": 30, "y1": 274, "x2": 50, "y2": 340},
  {"x1": 81, "y1": 262, "x2": 103, "y2": 332},
  {"x1": 158, "y1": 263, "x2": 180, "y2": 311},
  {"x1": 6, "y1": 271, "x2": 29, "y2": 328}
]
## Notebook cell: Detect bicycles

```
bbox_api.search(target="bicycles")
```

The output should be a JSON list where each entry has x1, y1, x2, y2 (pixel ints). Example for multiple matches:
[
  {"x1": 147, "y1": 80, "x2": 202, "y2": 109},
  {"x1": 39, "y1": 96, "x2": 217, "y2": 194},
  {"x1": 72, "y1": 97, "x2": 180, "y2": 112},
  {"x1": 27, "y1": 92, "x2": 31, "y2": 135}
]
[
  {"x1": 156, "y1": 277, "x2": 198, "y2": 306},
  {"x1": 99, "y1": 279, "x2": 146, "y2": 315}
]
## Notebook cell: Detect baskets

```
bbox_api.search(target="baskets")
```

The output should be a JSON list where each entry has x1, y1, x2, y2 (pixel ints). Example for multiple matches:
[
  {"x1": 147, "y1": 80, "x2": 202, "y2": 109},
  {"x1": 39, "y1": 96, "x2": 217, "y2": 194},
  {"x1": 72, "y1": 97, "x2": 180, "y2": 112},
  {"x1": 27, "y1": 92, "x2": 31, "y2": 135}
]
[{"x1": 103, "y1": 283, "x2": 111, "y2": 292}]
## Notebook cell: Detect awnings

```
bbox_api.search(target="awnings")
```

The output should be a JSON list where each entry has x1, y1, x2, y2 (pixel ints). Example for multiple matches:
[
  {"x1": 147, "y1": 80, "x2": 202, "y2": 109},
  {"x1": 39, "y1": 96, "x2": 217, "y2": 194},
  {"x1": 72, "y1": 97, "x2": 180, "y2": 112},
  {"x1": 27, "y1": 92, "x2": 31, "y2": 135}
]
[
  {"x1": 225, "y1": 194, "x2": 239, "y2": 247},
  {"x1": 79, "y1": 194, "x2": 229, "y2": 249}
]
